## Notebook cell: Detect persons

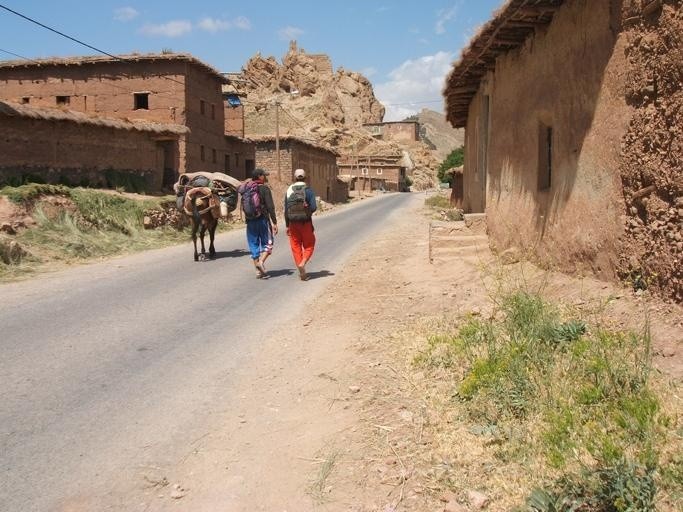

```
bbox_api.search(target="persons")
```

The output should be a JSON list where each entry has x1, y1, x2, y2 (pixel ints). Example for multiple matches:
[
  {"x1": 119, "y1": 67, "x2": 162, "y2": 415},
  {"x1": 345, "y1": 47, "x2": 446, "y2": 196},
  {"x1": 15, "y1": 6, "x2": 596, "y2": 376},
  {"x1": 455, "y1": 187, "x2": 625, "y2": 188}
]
[
  {"x1": 245, "y1": 169, "x2": 277, "y2": 279},
  {"x1": 284, "y1": 169, "x2": 317, "y2": 281}
]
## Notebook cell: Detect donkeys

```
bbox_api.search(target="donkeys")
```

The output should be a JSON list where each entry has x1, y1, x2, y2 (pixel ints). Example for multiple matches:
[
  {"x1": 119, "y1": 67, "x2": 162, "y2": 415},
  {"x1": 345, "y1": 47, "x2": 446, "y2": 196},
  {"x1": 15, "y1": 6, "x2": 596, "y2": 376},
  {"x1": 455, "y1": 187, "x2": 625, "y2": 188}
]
[{"x1": 181, "y1": 188, "x2": 220, "y2": 263}]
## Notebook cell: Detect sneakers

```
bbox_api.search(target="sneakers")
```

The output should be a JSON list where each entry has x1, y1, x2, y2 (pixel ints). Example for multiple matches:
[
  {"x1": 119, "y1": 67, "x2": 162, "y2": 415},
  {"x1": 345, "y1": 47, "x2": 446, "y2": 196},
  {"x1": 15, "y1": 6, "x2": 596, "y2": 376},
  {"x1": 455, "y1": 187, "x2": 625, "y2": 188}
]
[
  {"x1": 256, "y1": 261, "x2": 266, "y2": 274},
  {"x1": 255, "y1": 272, "x2": 268, "y2": 279}
]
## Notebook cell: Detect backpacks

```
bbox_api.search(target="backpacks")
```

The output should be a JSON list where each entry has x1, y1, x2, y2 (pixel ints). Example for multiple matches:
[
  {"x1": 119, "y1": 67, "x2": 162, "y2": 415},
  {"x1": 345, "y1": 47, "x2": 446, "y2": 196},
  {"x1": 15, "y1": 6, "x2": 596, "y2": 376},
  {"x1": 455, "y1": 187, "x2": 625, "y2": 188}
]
[
  {"x1": 287, "y1": 185, "x2": 312, "y2": 222},
  {"x1": 238, "y1": 179, "x2": 264, "y2": 220}
]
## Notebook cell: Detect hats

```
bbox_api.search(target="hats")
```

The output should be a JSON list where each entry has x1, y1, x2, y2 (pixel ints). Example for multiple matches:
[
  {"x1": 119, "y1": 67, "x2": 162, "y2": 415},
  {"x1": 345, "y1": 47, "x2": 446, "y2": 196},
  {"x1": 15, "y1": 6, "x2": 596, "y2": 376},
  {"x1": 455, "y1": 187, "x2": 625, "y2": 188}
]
[
  {"x1": 294, "y1": 168, "x2": 307, "y2": 180},
  {"x1": 252, "y1": 168, "x2": 270, "y2": 178}
]
